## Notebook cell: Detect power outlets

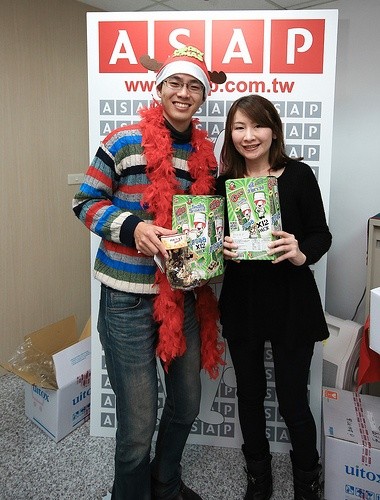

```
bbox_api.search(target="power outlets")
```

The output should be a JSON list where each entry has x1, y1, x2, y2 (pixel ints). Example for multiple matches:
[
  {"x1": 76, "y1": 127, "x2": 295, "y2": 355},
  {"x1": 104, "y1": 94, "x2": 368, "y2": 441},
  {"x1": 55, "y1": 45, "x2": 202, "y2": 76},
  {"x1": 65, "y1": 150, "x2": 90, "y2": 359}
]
[{"x1": 68, "y1": 173, "x2": 85, "y2": 185}]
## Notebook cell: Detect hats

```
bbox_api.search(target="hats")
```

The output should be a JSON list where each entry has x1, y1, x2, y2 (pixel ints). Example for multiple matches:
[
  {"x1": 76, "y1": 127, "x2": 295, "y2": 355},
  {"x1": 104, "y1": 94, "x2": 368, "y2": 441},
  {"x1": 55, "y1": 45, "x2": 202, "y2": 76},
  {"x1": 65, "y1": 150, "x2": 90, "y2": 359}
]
[{"x1": 140, "y1": 45, "x2": 226, "y2": 107}]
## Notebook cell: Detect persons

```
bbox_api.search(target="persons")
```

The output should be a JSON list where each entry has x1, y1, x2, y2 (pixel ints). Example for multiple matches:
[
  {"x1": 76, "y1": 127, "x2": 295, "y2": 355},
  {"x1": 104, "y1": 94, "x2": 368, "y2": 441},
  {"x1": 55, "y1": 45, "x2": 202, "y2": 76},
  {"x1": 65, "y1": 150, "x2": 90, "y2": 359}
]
[
  {"x1": 72, "y1": 45, "x2": 227, "y2": 500},
  {"x1": 215, "y1": 95, "x2": 332, "y2": 500}
]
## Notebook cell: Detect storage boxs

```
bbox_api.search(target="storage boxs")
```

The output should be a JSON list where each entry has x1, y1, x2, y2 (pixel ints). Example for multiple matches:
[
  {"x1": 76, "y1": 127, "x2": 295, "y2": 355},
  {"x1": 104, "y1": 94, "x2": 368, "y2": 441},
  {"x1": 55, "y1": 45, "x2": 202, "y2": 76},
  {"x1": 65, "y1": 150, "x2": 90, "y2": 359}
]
[
  {"x1": 171, "y1": 194, "x2": 225, "y2": 280},
  {"x1": 226, "y1": 176, "x2": 285, "y2": 260},
  {"x1": 322, "y1": 387, "x2": 380, "y2": 500},
  {"x1": 1, "y1": 314, "x2": 92, "y2": 444}
]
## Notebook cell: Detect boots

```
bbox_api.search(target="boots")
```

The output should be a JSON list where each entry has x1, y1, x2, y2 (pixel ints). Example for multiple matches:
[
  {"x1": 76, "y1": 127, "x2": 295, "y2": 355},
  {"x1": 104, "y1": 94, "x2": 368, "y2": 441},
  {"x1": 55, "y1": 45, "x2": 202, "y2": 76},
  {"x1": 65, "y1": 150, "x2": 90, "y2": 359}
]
[
  {"x1": 289, "y1": 449, "x2": 323, "y2": 500},
  {"x1": 241, "y1": 443, "x2": 273, "y2": 500}
]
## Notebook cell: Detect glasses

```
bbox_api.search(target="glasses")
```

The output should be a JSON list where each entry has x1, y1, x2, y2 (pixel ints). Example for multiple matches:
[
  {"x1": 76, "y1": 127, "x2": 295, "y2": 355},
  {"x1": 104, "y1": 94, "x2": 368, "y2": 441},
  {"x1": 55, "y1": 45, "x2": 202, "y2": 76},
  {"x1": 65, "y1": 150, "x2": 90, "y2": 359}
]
[{"x1": 164, "y1": 78, "x2": 205, "y2": 93}]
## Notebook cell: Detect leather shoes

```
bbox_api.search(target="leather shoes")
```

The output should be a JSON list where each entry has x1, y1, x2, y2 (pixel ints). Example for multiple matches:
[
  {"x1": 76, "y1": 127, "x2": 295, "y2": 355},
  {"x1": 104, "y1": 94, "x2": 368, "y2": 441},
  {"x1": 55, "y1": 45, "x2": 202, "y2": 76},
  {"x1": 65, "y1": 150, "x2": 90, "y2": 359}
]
[{"x1": 154, "y1": 477, "x2": 203, "y2": 500}]
